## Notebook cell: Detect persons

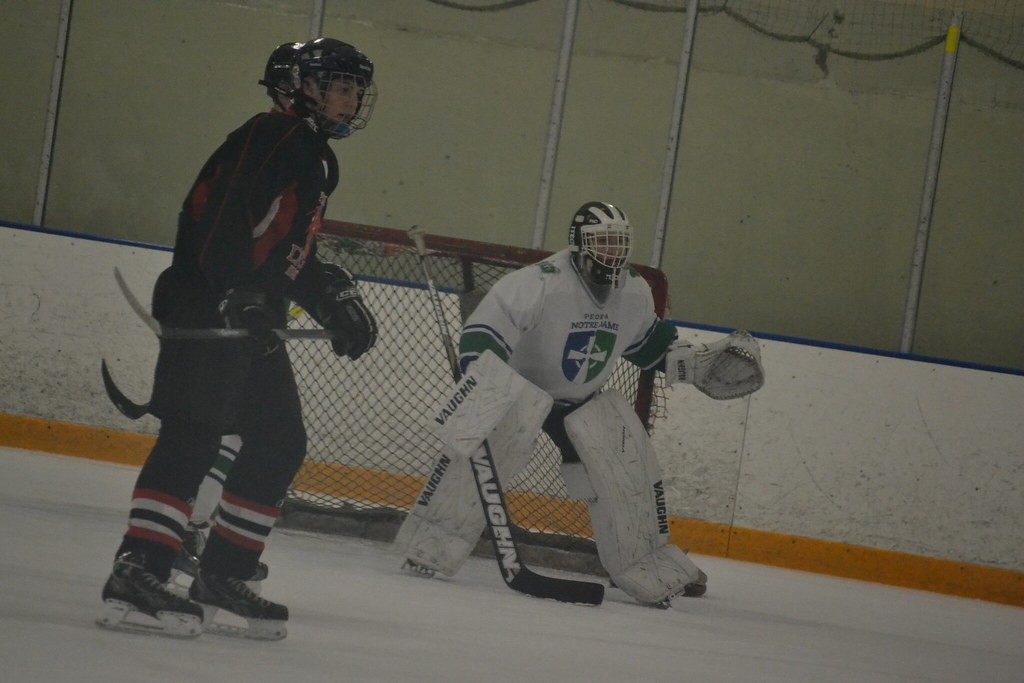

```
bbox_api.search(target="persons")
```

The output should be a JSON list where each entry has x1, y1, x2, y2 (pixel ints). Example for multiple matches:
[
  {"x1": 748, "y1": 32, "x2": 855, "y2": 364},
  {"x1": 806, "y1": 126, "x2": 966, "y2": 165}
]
[
  {"x1": 94, "y1": 37, "x2": 380, "y2": 639},
  {"x1": 388, "y1": 201, "x2": 766, "y2": 609}
]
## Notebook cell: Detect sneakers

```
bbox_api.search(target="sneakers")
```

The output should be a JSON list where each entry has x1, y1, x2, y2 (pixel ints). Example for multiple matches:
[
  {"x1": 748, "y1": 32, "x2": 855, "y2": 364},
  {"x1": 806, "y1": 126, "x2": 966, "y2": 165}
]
[
  {"x1": 240, "y1": 561, "x2": 268, "y2": 597},
  {"x1": 96, "y1": 546, "x2": 204, "y2": 638},
  {"x1": 187, "y1": 565, "x2": 289, "y2": 641},
  {"x1": 169, "y1": 520, "x2": 210, "y2": 588}
]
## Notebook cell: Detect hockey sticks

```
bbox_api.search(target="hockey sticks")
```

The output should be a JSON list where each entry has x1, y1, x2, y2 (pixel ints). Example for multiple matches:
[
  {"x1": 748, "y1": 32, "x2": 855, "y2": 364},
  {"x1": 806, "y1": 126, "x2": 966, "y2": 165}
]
[
  {"x1": 407, "y1": 224, "x2": 606, "y2": 605},
  {"x1": 113, "y1": 263, "x2": 328, "y2": 340},
  {"x1": 100, "y1": 357, "x2": 151, "y2": 422}
]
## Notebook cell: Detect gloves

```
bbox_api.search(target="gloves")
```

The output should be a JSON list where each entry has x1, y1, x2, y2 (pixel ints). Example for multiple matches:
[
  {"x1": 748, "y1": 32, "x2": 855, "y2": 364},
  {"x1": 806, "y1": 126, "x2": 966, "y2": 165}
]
[
  {"x1": 299, "y1": 263, "x2": 378, "y2": 360},
  {"x1": 203, "y1": 299, "x2": 272, "y2": 362}
]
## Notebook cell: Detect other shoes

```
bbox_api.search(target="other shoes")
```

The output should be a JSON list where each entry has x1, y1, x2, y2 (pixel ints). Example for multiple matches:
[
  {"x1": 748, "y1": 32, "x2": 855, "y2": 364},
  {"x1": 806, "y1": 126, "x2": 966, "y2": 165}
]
[
  {"x1": 681, "y1": 567, "x2": 708, "y2": 597},
  {"x1": 401, "y1": 558, "x2": 436, "y2": 579}
]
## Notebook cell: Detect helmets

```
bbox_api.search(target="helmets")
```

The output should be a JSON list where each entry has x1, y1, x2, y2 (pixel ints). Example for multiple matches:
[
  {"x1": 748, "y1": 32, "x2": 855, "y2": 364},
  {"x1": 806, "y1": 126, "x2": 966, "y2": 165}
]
[
  {"x1": 290, "y1": 38, "x2": 379, "y2": 139},
  {"x1": 569, "y1": 202, "x2": 632, "y2": 287},
  {"x1": 258, "y1": 42, "x2": 306, "y2": 97}
]
